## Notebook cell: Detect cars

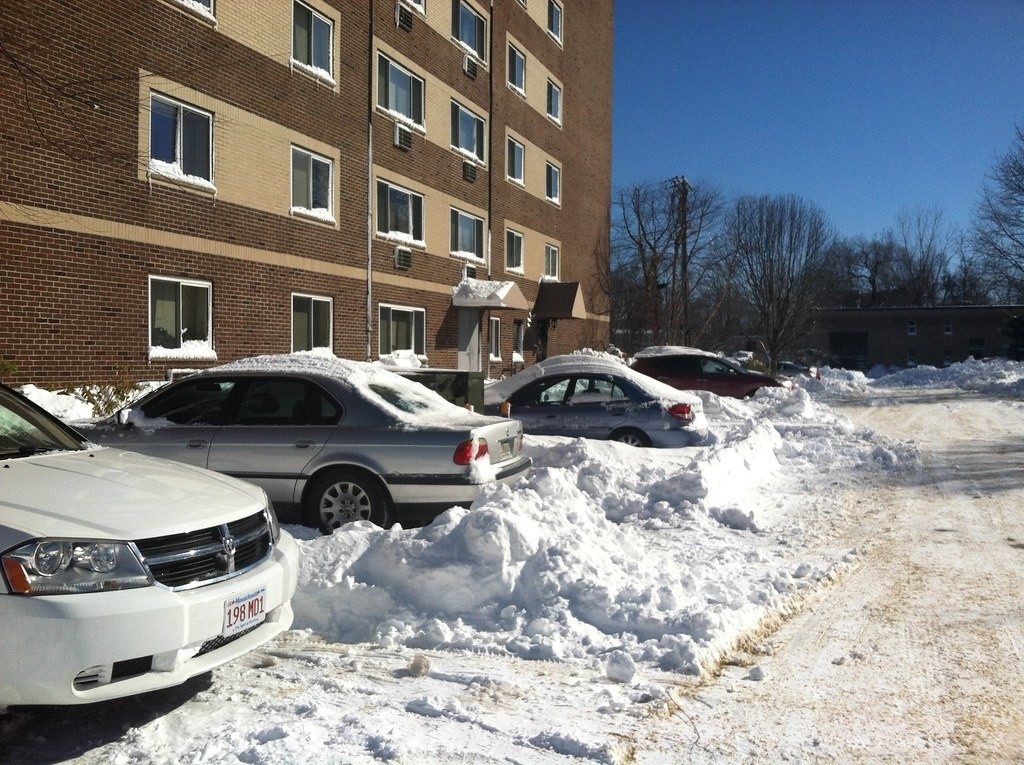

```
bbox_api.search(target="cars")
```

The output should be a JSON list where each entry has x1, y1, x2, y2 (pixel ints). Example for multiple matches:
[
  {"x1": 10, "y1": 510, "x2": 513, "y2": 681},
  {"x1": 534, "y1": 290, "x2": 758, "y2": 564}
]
[
  {"x1": 1, "y1": 384, "x2": 302, "y2": 706},
  {"x1": 776, "y1": 359, "x2": 821, "y2": 379},
  {"x1": 58, "y1": 351, "x2": 534, "y2": 535},
  {"x1": 628, "y1": 347, "x2": 787, "y2": 399},
  {"x1": 484, "y1": 354, "x2": 710, "y2": 447}
]
